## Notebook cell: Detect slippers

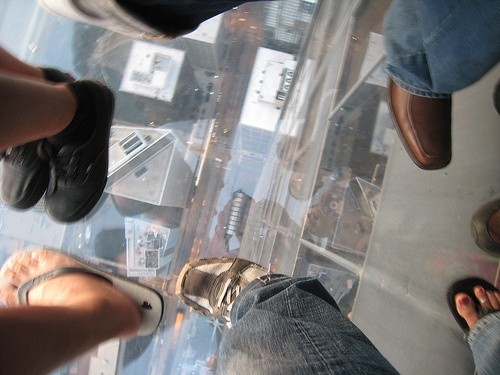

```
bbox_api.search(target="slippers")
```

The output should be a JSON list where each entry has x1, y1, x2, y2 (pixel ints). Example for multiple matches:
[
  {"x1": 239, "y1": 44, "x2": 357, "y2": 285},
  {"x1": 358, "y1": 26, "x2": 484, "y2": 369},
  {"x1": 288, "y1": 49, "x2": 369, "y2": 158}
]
[{"x1": 0, "y1": 245, "x2": 164, "y2": 337}]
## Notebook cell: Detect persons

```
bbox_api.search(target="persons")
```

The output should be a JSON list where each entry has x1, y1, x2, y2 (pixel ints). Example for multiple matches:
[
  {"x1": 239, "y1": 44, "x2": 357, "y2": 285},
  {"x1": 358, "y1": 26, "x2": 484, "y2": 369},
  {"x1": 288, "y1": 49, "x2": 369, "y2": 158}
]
[
  {"x1": 0, "y1": 46, "x2": 115, "y2": 225},
  {"x1": 35, "y1": 0, "x2": 260, "y2": 43},
  {"x1": 382, "y1": 0, "x2": 500, "y2": 171},
  {"x1": 176, "y1": 257, "x2": 401, "y2": 375},
  {"x1": 446, "y1": 199, "x2": 500, "y2": 375},
  {"x1": 0, "y1": 246, "x2": 166, "y2": 375}
]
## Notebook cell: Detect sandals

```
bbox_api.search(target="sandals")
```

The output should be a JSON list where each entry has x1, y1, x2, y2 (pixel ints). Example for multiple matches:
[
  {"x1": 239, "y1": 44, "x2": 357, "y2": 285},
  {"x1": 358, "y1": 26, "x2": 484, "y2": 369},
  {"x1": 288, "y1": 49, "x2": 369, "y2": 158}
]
[{"x1": 446, "y1": 275, "x2": 500, "y2": 338}]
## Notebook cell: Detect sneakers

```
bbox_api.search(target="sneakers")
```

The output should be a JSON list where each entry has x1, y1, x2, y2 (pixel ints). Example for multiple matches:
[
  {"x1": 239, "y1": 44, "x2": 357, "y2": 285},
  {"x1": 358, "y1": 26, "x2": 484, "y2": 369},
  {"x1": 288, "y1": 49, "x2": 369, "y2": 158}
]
[
  {"x1": 36, "y1": 78, "x2": 116, "y2": 225},
  {"x1": 173, "y1": 256, "x2": 282, "y2": 330},
  {"x1": 0, "y1": 67, "x2": 76, "y2": 213}
]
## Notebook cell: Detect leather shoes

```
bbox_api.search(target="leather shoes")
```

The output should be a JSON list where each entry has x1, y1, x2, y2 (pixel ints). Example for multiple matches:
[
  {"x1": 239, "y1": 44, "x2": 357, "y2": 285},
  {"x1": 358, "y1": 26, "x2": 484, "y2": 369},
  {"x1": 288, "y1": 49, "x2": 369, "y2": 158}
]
[{"x1": 387, "y1": 76, "x2": 453, "y2": 171}]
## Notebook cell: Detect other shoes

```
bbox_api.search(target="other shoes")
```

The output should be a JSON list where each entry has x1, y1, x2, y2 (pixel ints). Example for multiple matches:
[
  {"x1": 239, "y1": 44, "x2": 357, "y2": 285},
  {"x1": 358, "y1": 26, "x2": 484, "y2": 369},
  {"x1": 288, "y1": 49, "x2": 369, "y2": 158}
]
[
  {"x1": 471, "y1": 198, "x2": 500, "y2": 255},
  {"x1": 41, "y1": 0, "x2": 199, "y2": 42}
]
